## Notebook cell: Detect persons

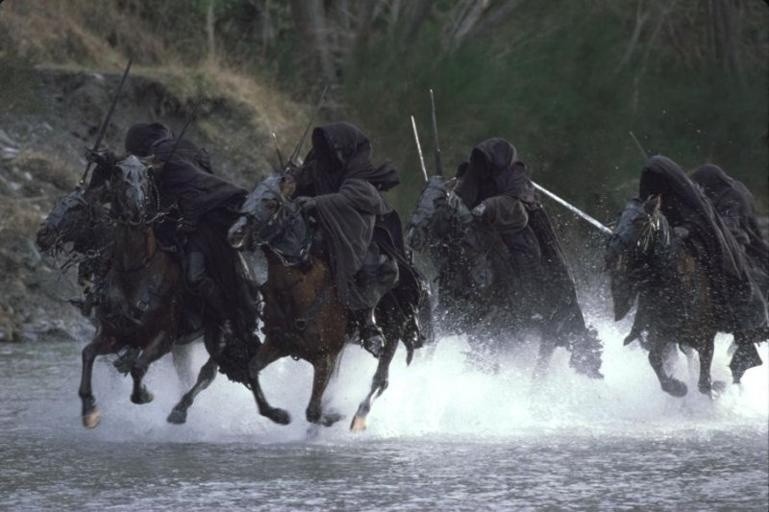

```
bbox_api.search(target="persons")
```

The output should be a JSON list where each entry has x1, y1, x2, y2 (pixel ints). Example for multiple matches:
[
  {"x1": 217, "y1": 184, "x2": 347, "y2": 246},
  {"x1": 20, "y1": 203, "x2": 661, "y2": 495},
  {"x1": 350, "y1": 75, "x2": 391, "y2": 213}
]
[
  {"x1": 634, "y1": 155, "x2": 768, "y2": 345},
  {"x1": 124, "y1": 120, "x2": 262, "y2": 332},
  {"x1": 450, "y1": 135, "x2": 585, "y2": 349},
  {"x1": 688, "y1": 162, "x2": 768, "y2": 268},
  {"x1": 280, "y1": 121, "x2": 401, "y2": 357}
]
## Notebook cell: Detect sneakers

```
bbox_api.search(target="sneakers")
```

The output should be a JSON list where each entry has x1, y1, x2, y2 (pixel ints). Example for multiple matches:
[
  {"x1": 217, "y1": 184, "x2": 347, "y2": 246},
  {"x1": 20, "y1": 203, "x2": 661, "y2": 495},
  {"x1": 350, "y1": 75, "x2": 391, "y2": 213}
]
[{"x1": 360, "y1": 318, "x2": 386, "y2": 358}]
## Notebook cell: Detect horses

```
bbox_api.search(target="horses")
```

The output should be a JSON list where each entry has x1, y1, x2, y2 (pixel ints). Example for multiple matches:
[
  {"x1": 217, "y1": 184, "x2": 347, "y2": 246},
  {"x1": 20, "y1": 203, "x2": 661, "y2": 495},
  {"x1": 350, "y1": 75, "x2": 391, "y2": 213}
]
[
  {"x1": 35, "y1": 150, "x2": 251, "y2": 428},
  {"x1": 611, "y1": 193, "x2": 769, "y2": 397},
  {"x1": 405, "y1": 169, "x2": 605, "y2": 395},
  {"x1": 170, "y1": 177, "x2": 430, "y2": 428}
]
